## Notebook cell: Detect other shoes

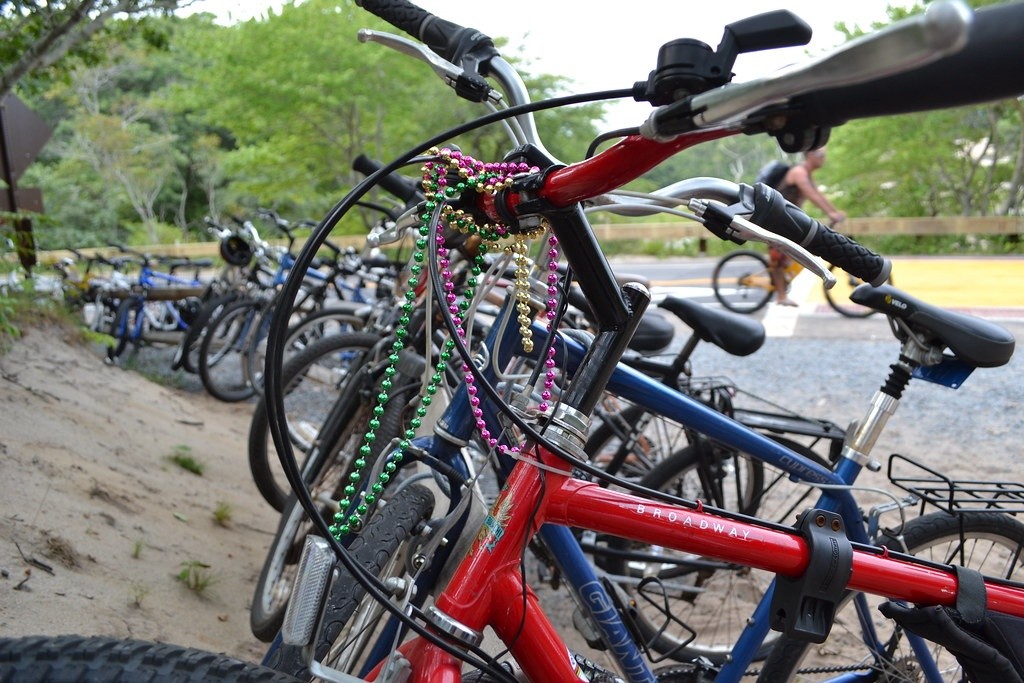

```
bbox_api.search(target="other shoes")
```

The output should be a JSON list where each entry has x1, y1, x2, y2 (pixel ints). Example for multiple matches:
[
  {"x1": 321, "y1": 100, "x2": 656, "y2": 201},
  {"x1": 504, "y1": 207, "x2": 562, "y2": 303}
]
[{"x1": 777, "y1": 298, "x2": 798, "y2": 307}]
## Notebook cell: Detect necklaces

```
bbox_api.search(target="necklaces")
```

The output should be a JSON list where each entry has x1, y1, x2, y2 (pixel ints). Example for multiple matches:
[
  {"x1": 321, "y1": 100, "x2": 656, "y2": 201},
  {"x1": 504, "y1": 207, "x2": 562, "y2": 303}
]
[{"x1": 309, "y1": 145, "x2": 559, "y2": 544}]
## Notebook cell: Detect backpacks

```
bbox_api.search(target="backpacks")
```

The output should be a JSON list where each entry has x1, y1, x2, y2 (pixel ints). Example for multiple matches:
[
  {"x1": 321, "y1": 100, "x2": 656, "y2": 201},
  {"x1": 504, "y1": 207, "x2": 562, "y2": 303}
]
[{"x1": 753, "y1": 161, "x2": 789, "y2": 186}]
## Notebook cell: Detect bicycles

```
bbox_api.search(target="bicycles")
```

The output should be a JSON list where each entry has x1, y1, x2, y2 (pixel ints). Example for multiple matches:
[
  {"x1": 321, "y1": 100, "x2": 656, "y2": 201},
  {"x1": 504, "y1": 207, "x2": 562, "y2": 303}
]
[{"x1": 36, "y1": 0, "x2": 1024, "y2": 683}]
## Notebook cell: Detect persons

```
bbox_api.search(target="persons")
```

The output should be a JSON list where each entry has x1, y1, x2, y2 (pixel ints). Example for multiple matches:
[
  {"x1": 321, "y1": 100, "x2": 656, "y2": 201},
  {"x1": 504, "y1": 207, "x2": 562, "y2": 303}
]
[{"x1": 765, "y1": 147, "x2": 845, "y2": 307}]
